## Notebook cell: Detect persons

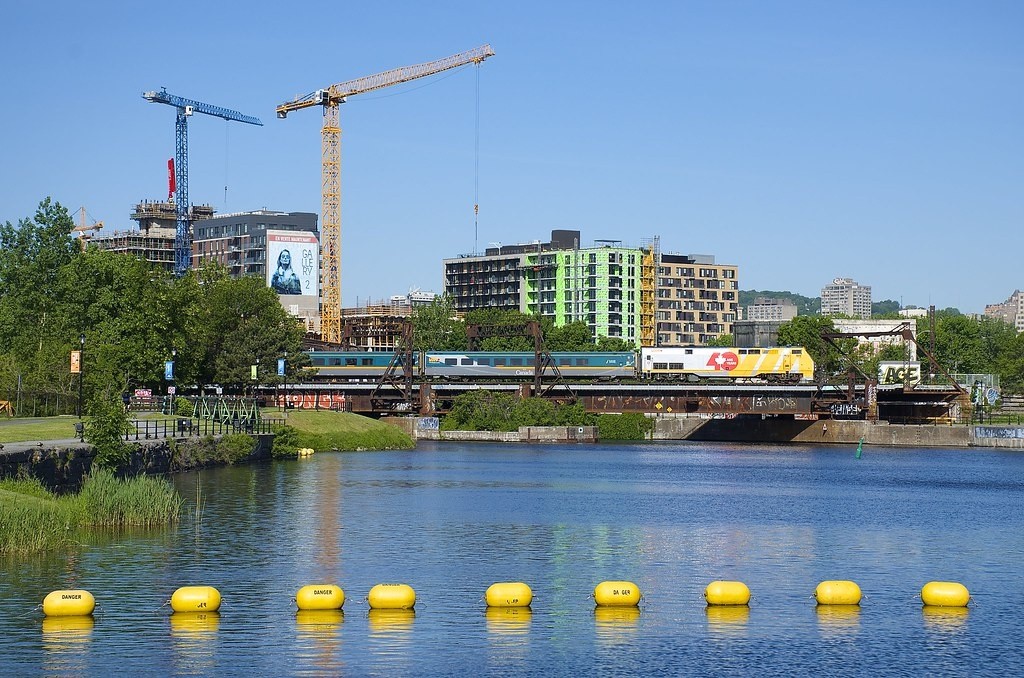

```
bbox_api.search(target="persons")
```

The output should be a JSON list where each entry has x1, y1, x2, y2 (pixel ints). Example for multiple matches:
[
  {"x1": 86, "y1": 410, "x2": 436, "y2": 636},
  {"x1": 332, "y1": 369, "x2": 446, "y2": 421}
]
[
  {"x1": 122, "y1": 390, "x2": 132, "y2": 408},
  {"x1": 272, "y1": 250, "x2": 302, "y2": 295}
]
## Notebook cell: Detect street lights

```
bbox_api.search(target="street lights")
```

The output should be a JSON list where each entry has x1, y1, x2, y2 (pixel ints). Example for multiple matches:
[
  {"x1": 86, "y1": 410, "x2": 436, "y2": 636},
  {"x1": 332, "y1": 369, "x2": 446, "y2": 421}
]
[
  {"x1": 171, "y1": 347, "x2": 177, "y2": 413},
  {"x1": 284, "y1": 349, "x2": 288, "y2": 414},
  {"x1": 255, "y1": 356, "x2": 260, "y2": 414},
  {"x1": 77, "y1": 333, "x2": 87, "y2": 421}
]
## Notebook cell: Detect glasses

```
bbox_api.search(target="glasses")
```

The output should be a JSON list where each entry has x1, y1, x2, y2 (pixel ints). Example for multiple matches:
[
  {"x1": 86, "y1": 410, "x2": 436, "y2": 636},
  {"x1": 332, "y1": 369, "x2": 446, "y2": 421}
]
[{"x1": 281, "y1": 254, "x2": 290, "y2": 259}]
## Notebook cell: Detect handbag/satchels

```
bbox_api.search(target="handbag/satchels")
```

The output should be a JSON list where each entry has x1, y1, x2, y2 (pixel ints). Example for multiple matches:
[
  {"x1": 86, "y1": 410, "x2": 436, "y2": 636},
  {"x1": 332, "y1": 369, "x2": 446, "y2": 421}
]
[{"x1": 128, "y1": 396, "x2": 133, "y2": 402}]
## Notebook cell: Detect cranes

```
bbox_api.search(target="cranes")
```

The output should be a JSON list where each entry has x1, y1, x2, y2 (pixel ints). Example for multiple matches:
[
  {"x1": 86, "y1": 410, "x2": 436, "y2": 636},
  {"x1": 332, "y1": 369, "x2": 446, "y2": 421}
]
[
  {"x1": 62, "y1": 205, "x2": 103, "y2": 248},
  {"x1": 277, "y1": 43, "x2": 494, "y2": 341},
  {"x1": 148, "y1": 86, "x2": 264, "y2": 280}
]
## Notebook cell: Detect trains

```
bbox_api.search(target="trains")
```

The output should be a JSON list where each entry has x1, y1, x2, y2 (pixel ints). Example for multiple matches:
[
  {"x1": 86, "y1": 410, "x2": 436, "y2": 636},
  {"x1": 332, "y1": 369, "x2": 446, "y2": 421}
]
[{"x1": 299, "y1": 346, "x2": 827, "y2": 385}]
